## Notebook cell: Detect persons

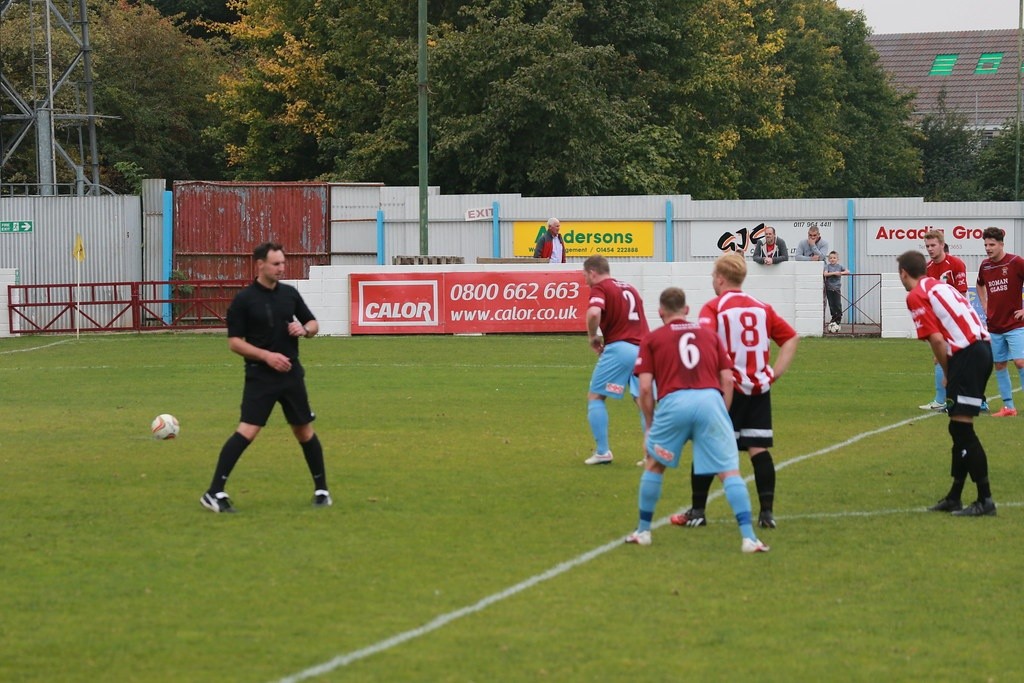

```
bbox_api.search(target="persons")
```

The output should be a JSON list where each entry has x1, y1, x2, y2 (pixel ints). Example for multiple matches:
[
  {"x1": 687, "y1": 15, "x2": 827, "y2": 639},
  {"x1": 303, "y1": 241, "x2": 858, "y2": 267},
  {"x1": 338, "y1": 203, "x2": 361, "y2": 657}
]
[
  {"x1": 670, "y1": 252, "x2": 800, "y2": 529},
  {"x1": 583, "y1": 254, "x2": 658, "y2": 467},
  {"x1": 625, "y1": 286, "x2": 771, "y2": 553},
  {"x1": 943, "y1": 242, "x2": 991, "y2": 413},
  {"x1": 976, "y1": 227, "x2": 1024, "y2": 416},
  {"x1": 753, "y1": 227, "x2": 789, "y2": 265},
  {"x1": 824, "y1": 250, "x2": 850, "y2": 333},
  {"x1": 533, "y1": 218, "x2": 566, "y2": 263},
  {"x1": 918, "y1": 229, "x2": 970, "y2": 409},
  {"x1": 199, "y1": 241, "x2": 332, "y2": 513},
  {"x1": 896, "y1": 250, "x2": 997, "y2": 516},
  {"x1": 796, "y1": 226, "x2": 828, "y2": 333}
]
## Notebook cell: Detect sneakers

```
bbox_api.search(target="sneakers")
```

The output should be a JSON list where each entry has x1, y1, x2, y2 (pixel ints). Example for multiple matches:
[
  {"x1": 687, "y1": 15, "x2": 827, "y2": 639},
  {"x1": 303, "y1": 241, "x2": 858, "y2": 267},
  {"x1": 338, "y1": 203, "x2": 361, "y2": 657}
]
[
  {"x1": 313, "y1": 489, "x2": 332, "y2": 507},
  {"x1": 953, "y1": 498, "x2": 997, "y2": 516},
  {"x1": 918, "y1": 398, "x2": 948, "y2": 411},
  {"x1": 939, "y1": 407, "x2": 948, "y2": 414},
  {"x1": 741, "y1": 537, "x2": 771, "y2": 552},
  {"x1": 990, "y1": 407, "x2": 1017, "y2": 417},
  {"x1": 926, "y1": 497, "x2": 962, "y2": 513},
  {"x1": 670, "y1": 509, "x2": 706, "y2": 527},
  {"x1": 980, "y1": 402, "x2": 989, "y2": 413},
  {"x1": 584, "y1": 451, "x2": 613, "y2": 466},
  {"x1": 625, "y1": 529, "x2": 652, "y2": 546},
  {"x1": 200, "y1": 490, "x2": 236, "y2": 514},
  {"x1": 758, "y1": 512, "x2": 776, "y2": 529},
  {"x1": 636, "y1": 459, "x2": 647, "y2": 465}
]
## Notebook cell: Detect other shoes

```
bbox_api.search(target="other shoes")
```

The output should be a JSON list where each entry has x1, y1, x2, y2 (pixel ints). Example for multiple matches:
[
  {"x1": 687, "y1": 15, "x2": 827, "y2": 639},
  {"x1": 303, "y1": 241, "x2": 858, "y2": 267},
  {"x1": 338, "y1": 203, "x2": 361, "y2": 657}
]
[{"x1": 830, "y1": 316, "x2": 841, "y2": 324}]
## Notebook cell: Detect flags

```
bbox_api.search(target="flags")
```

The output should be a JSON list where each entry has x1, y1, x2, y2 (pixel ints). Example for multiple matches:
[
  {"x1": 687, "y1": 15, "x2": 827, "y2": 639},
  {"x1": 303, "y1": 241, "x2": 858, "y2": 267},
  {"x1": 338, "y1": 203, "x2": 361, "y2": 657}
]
[{"x1": 73, "y1": 235, "x2": 87, "y2": 262}]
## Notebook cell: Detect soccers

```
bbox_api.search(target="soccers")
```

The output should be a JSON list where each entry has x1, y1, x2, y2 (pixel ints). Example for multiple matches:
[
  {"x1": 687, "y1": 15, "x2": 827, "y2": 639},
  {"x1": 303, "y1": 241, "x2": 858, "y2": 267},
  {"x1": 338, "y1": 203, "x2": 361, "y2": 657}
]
[
  {"x1": 151, "y1": 413, "x2": 180, "y2": 439},
  {"x1": 828, "y1": 322, "x2": 841, "y2": 333}
]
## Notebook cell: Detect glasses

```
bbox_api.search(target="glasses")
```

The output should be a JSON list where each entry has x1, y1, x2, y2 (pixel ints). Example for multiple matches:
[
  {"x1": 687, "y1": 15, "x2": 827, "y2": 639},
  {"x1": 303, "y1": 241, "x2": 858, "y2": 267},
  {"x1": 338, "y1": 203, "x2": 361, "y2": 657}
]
[{"x1": 809, "y1": 234, "x2": 818, "y2": 236}]
